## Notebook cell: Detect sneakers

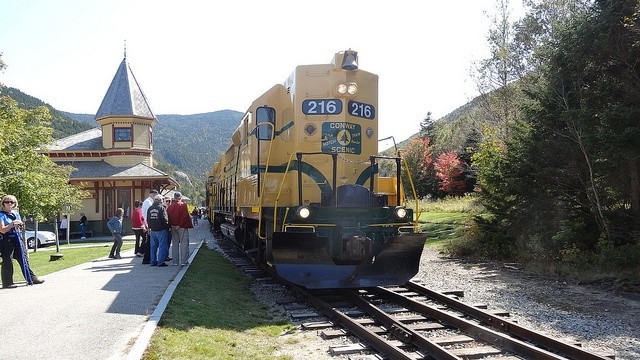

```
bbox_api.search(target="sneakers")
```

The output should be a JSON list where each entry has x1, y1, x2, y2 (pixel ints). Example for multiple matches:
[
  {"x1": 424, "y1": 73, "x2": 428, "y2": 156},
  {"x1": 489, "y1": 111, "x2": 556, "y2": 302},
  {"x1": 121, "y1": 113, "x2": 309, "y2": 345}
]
[
  {"x1": 164, "y1": 257, "x2": 172, "y2": 261},
  {"x1": 31, "y1": 276, "x2": 45, "y2": 284},
  {"x1": 3, "y1": 284, "x2": 17, "y2": 288},
  {"x1": 181, "y1": 261, "x2": 191, "y2": 266},
  {"x1": 174, "y1": 264, "x2": 178, "y2": 265},
  {"x1": 81, "y1": 236, "x2": 86, "y2": 240}
]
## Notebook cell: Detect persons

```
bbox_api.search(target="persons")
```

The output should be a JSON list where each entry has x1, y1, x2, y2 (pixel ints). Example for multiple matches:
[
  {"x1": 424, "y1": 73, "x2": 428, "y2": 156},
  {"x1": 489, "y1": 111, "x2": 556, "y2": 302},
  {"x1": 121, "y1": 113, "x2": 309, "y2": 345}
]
[
  {"x1": 132, "y1": 201, "x2": 147, "y2": 257},
  {"x1": 146, "y1": 195, "x2": 168, "y2": 266},
  {"x1": 167, "y1": 192, "x2": 192, "y2": 266},
  {"x1": 79, "y1": 213, "x2": 88, "y2": 240},
  {"x1": 60, "y1": 215, "x2": 67, "y2": 240},
  {"x1": 142, "y1": 190, "x2": 158, "y2": 264},
  {"x1": 191, "y1": 207, "x2": 199, "y2": 226},
  {"x1": 0, "y1": 195, "x2": 44, "y2": 288},
  {"x1": 107, "y1": 208, "x2": 123, "y2": 259}
]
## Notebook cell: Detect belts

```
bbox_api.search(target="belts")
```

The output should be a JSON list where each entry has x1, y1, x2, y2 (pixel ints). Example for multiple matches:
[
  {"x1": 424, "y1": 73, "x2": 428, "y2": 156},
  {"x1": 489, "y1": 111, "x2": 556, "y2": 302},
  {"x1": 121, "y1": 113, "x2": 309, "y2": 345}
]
[
  {"x1": 3, "y1": 235, "x2": 16, "y2": 237},
  {"x1": 114, "y1": 233, "x2": 121, "y2": 234}
]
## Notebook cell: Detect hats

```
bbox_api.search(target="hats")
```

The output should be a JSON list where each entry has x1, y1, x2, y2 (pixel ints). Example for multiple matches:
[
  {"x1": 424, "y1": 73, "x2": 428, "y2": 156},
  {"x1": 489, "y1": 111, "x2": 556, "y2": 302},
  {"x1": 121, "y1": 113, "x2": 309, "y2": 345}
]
[
  {"x1": 174, "y1": 192, "x2": 183, "y2": 198},
  {"x1": 154, "y1": 195, "x2": 165, "y2": 201}
]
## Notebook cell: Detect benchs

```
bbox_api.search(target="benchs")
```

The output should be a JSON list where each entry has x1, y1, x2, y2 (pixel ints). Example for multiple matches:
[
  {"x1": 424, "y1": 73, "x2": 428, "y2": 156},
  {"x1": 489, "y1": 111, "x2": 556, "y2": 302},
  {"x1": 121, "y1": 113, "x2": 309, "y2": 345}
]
[{"x1": 59, "y1": 230, "x2": 94, "y2": 238}]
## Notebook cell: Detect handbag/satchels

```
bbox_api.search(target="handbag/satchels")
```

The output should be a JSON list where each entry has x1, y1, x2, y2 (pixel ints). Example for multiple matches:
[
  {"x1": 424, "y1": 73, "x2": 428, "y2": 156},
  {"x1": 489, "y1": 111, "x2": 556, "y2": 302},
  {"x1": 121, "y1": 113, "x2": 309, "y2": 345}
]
[{"x1": 85, "y1": 220, "x2": 88, "y2": 225}]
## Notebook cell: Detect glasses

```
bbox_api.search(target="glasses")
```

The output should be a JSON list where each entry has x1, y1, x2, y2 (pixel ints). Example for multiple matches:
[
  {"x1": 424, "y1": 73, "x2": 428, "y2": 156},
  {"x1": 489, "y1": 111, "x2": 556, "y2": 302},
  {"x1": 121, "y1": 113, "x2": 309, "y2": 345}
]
[{"x1": 4, "y1": 201, "x2": 15, "y2": 204}]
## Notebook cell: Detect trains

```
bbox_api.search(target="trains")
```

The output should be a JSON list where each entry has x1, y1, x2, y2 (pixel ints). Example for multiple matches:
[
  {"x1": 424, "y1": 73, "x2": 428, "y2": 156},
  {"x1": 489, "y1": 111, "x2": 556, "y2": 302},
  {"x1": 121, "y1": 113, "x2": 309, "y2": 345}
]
[{"x1": 205, "y1": 49, "x2": 428, "y2": 289}]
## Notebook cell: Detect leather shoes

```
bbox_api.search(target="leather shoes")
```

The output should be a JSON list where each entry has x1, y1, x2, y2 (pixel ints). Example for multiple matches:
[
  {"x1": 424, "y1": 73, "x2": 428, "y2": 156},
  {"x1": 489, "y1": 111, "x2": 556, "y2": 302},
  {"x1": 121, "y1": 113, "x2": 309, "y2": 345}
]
[
  {"x1": 158, "y1": 263, "x2": 168, "y2": 266},
  {"x1": 151, "y1": 262, "x2": 158, "y2": 266}
]
